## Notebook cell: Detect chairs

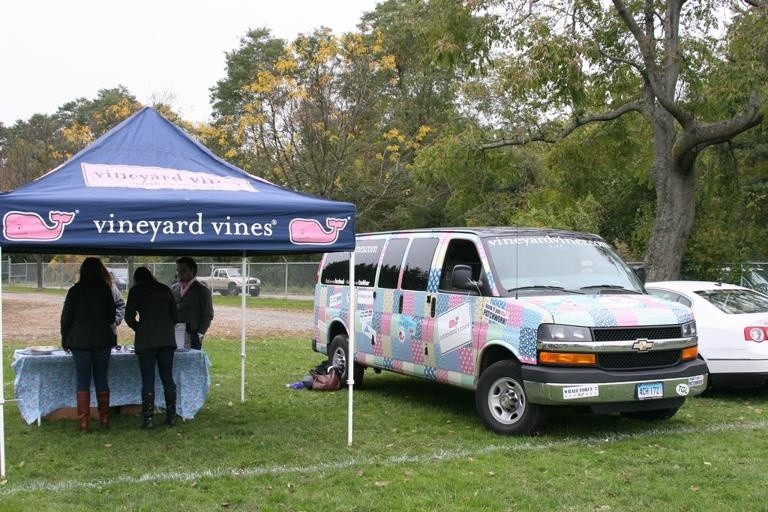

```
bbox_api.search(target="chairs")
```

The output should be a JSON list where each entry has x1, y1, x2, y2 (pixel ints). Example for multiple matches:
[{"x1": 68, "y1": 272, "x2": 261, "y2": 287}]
[{"x1": 444, "y1": 258, "x2": 477, "y2": 294}]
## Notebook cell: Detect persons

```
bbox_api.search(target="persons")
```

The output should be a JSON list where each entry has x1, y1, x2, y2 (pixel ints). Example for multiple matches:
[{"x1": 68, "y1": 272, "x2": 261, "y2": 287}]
[
  {"x1": 109, "y1": 282, "x2": 126, "y2": 348},
  {"x1": 59, "y1": 257, "x2": 116, "y2": 434},
  {"x1": 124, "y1": 266, "x2": 178, "y2": 430},
  {"x1": 169, "y1": 257, "x2": 214, "y2": 351}
]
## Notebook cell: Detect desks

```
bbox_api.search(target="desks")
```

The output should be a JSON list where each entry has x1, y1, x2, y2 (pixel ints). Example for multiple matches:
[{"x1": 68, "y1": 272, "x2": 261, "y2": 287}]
[{"x1": 11, "y1": 345, "x2": 213, "y2": 426}]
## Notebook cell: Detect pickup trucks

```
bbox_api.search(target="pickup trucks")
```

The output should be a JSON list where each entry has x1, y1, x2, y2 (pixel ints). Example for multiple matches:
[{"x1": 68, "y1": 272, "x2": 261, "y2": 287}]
[{"x1": 185, "y1": 267, "x2": 261, "y2": 296}]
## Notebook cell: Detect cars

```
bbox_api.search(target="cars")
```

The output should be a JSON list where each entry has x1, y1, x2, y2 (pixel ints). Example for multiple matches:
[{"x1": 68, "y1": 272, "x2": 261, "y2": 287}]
[
  {"x1": 108, "y1": 268, "x2": 127, "y2": 290},
  {"x1": 643, "y1": 278, "x2": 767, "y2": 398}
]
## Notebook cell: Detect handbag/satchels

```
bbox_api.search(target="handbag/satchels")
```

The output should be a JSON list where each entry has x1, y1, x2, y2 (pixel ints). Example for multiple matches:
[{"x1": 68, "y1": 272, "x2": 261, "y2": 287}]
[{"x1": 312, "y1": 370, "x2": 340, "y2": 391}]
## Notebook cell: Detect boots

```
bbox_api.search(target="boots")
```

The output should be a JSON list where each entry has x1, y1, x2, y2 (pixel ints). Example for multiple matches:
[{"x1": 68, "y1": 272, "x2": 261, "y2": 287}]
[
  {"x1": 78, "y1": 391, "x2": 90, "y2": 432},
  {"x1": 142, "y1": 390, "x2": 154, "y2": 429},
  {"x1": 163, "y1": 385, "x2": 176, "y2": 425},
  {"x1": 96, "y1": 390, "x2": 110, "y2": 430}
]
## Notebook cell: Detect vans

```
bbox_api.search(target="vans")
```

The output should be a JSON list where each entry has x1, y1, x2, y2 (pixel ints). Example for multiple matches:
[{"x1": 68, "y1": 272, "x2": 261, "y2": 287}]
[{"x1": 309, "y1": 224, "x2": 709, "y2": 435}]
